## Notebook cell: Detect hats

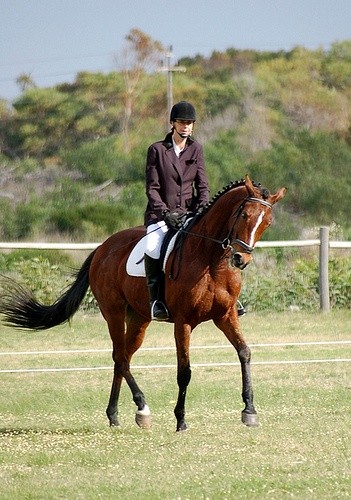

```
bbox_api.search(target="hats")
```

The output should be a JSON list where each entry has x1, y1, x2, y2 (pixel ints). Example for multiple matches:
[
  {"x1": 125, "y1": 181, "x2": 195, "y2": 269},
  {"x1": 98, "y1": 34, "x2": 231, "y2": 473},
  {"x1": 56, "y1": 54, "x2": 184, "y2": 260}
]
[{"x1": 169, "y1": 100, "x2": 196, "y2": 122}]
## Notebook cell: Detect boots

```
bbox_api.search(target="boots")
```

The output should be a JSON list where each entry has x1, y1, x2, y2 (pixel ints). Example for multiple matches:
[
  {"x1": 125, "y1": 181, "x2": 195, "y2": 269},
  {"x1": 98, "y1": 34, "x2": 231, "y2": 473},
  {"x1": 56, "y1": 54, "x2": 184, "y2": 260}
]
[{"x1": 143, "y1": 252, "x2": 168, "y2": 320}]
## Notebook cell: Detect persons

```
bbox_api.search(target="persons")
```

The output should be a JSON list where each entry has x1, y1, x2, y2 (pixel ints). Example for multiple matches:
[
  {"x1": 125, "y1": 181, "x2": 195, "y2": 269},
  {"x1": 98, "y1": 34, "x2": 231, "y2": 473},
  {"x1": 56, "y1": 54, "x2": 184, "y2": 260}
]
[{"x1": 145, "y1": 101, "x2": 246, "y2": 322}]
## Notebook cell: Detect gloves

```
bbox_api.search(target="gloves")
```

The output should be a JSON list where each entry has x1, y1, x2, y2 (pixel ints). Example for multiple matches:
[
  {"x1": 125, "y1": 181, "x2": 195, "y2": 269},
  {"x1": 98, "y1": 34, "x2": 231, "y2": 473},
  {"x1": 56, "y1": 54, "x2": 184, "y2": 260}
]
[{"x1": 164, "y1": 212, "x2": 180, "y2": 231}]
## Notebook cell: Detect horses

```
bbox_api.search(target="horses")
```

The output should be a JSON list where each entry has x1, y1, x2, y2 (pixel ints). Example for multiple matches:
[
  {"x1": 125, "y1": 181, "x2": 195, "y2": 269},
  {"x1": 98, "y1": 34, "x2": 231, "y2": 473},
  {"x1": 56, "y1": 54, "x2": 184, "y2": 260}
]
[{"x1": 0, "y1": 174, "x2": 288, "y2": 434}]
